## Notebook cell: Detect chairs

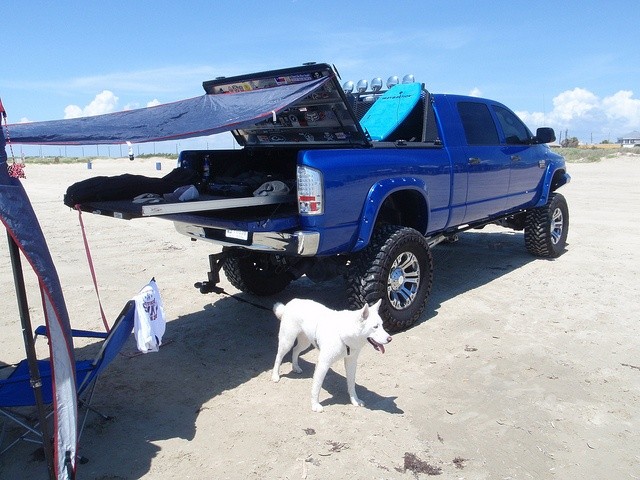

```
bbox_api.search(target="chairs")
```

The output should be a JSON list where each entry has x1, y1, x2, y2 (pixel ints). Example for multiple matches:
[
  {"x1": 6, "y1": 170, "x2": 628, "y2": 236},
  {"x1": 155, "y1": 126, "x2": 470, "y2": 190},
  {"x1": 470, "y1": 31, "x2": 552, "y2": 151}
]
[{"x1": 0, "y1": 277, "x2": 157, "y2": 463}]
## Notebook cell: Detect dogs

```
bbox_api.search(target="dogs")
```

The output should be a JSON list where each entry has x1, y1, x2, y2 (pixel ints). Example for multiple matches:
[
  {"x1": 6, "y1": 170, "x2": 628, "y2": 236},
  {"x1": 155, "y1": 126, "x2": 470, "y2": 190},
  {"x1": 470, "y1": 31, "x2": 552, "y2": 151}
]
[{"x1": 270, "y1": 298, "x2": 393, "y2": 411}]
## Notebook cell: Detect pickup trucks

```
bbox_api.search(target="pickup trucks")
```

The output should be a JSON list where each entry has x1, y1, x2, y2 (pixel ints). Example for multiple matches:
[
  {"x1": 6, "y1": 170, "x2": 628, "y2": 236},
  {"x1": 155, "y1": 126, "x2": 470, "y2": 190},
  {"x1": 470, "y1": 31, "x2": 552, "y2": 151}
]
[{"x1": 173, "y1": 62, "x2": 571, "y2": 331}]
